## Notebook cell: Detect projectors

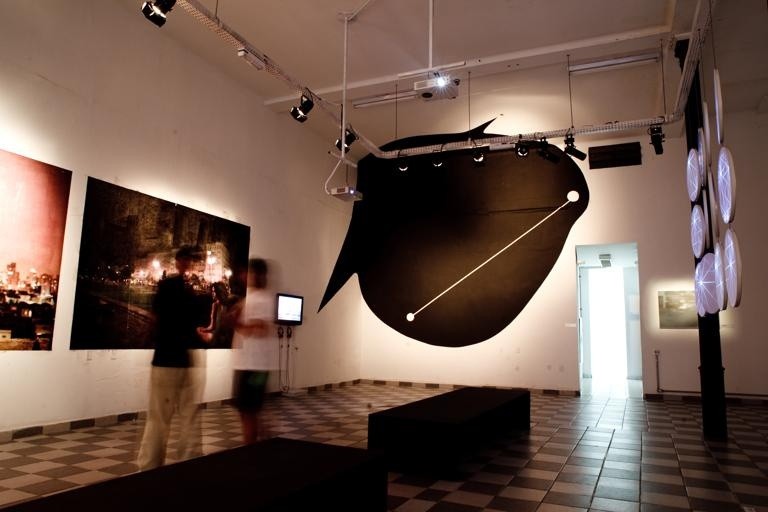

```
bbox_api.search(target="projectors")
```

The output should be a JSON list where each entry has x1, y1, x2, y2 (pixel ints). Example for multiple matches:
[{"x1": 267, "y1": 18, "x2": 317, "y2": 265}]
[
  {"x1": 413, "y1": 76, "x2": 459, "y2": 102},
  {"x1": 330, "y1": 187, "x2": 363, "y2": 202}
]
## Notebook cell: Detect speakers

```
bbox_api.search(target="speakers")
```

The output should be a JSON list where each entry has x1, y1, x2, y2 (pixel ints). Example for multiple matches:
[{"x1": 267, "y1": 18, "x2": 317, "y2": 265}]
[{"x1": 587, "y1": 141, "x2": 642, "y2": 169}]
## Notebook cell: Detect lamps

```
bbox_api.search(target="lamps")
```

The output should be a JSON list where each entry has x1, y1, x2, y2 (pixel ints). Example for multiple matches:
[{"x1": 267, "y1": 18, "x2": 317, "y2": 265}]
[
  {"x1": 395, "y1": 153, "x2": 411, "y2": 174},
  {"x1": 287, "y1": 92, "x2": 317, "y2": 125},
  {"x1": 140, "y1": 1, "x2": 177, "y2": 29},
  {"x1": 646, "y1": 123, "x2": 668, "y2": 156},
  {"x1": 333, "y1": 122, "x2": 360, "y2": 155},
  {"x1": 537, "y1": 136, "x2": 561, "y2": 164},
  {"x1": 516, "y1": 146, "x2": 530, "y2": 157},
  {"x1": 564, "y1": 134, "x2": 588, "y2": 162},
  {"x1": 431, "y1": 148, "x2": 445, "y2": 169},
  {"x1": 472, "y1": 151, "x2": 486, "y2": 163}
]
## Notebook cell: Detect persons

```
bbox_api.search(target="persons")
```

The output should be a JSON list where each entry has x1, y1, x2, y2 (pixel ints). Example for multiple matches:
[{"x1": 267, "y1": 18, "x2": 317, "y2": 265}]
[
  {"x1": 135, "y1": 245, "x2": 217, "y2": 472},
  {"x1": 198, "y1": 280, "x2": 229, "y2": 340},
  {"x1": 219, "y1": 259, "x2": 278, "y2": 450},
  {"x1": 207, "y1": 273, "x2": 245, "y2": 348}
]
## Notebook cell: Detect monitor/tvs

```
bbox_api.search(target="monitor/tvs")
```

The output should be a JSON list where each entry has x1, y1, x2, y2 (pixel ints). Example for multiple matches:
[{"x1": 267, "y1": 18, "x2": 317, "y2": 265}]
[{"x1": 275, "y1": 292, "x2": 304, "y2": 326}]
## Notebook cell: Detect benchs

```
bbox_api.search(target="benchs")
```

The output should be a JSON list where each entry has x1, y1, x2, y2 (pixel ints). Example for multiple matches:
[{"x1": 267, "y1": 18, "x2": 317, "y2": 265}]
[
  {"x1": 0, "y1": 437, "x2": 389, "y2": 512},
  {"x1": 368, "y1": 386, "x2": 531, "y2": 481}
]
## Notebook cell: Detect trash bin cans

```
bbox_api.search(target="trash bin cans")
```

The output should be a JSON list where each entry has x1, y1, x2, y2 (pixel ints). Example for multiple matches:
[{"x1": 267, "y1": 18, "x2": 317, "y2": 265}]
[{"x1": 233, "y1": 369, "x2": 268, "y2": 410}]
